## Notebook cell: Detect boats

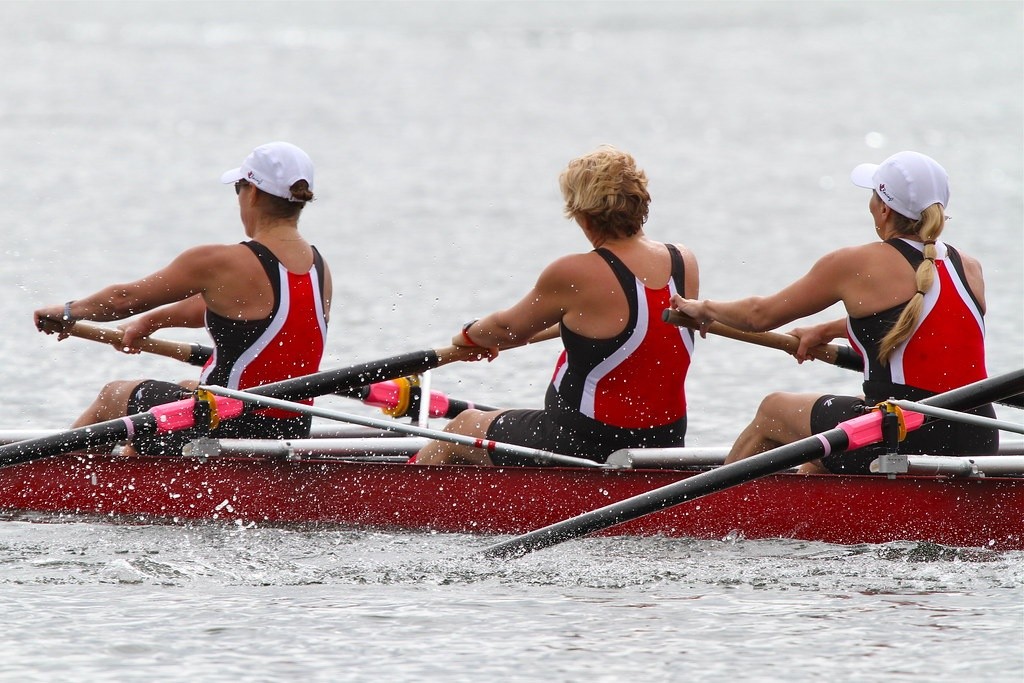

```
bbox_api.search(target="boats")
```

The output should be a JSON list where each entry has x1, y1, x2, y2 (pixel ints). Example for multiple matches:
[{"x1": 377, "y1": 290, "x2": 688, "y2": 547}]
[{"x1": 0, "y1": 431, "x2": 1024, "y2": 549}]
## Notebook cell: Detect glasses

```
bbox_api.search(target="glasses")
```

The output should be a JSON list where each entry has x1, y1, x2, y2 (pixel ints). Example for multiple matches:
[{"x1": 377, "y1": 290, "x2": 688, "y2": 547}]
[{"x1": 235, "y1": 182, "x2": 249, "y2": 195}]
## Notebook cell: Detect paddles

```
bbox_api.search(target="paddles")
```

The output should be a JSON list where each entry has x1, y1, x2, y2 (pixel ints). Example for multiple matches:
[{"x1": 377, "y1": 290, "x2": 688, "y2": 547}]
[
  {"x1": 660, "y1": 307, "x2": 1023, "y2": 411},
  {"x1": 1, "y1": 345, "x2": 493, "y2": 470},
  {"x1": 474, "y1": 367, "x2": 1024, "y2": 562},
  {"x1": 39, "y1": 316, "x2": 506, "y2": 420}
]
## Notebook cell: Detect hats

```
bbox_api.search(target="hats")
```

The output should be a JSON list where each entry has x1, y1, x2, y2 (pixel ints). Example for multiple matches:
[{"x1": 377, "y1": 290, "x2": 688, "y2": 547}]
[
  {"x1": 221, "y1": 141, "x2": 317, "y2": 202},
  {"x1": 850, "y1": 151, "x2": 952, "y2": 221}
]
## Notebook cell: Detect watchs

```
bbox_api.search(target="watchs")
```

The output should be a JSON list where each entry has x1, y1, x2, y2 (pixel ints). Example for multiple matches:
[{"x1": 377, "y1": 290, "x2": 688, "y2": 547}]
[
  {"x1": 463, "y1": 318, "x2": 481, "y2": 348},
  {"x1": 64, "y1": 300, "x2": 83, "y2": 321}
]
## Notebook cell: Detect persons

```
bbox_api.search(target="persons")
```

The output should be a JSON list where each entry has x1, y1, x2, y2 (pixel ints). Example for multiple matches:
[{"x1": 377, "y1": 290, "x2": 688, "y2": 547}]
[
  {"x1": 34, "y1": 141, "x2": 332, "y2": 459},
  {"x1": 671, "y1": 151, "x2": 1000, "y2": 473},
  {"x1": 405, "y1": 144, "x2": 700, "y2": 467}
]
